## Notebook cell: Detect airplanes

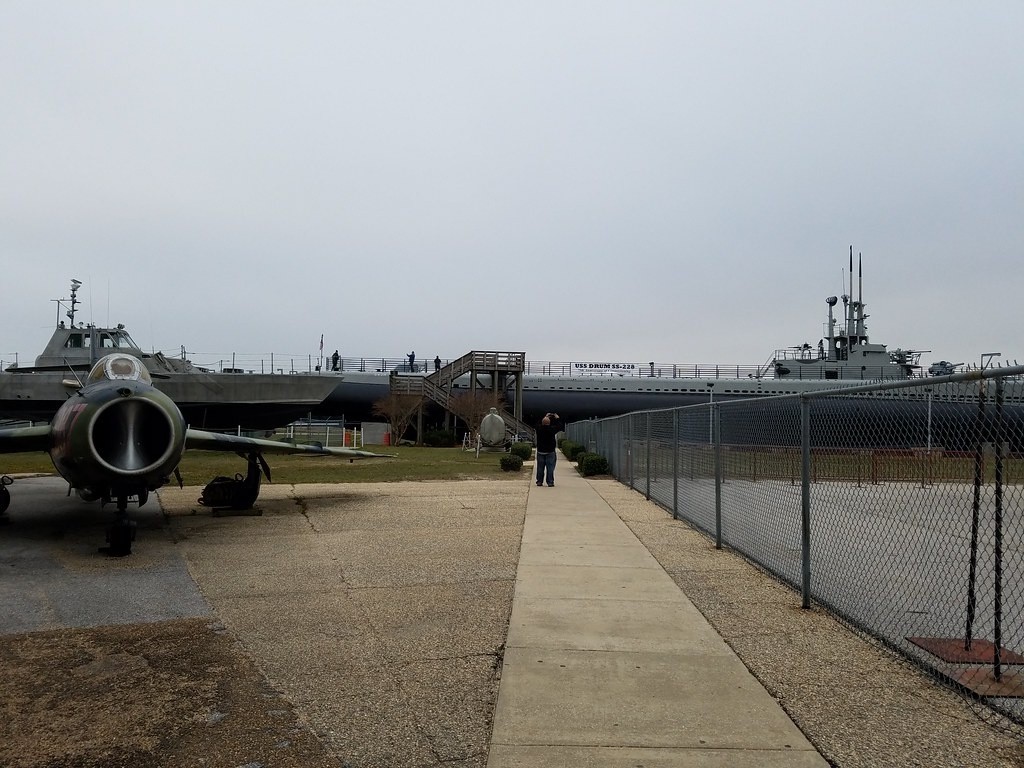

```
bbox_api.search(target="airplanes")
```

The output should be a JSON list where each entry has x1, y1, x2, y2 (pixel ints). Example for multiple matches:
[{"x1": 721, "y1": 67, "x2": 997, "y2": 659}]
[{"x1": 0, "y1": 351, "x2": 400, "y2": 555}]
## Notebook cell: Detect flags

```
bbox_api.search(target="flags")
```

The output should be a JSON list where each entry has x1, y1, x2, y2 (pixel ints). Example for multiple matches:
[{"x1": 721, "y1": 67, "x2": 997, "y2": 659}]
[{"x1": 320, "y1": 336, "x2": 323, "y2": 350}]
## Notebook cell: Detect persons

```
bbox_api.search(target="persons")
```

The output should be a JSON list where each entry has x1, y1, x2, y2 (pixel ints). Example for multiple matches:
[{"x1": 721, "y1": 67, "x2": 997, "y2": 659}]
[
  {"x1": 535, "y1": 413, "x2": 566, "y2": 487},
  {"x1": 434, "y1": 356, "x2": 441, "y2": 372},
  {"x1": 406, "y1": 350, "x2": 415, "y2": 372},
  {"x1": 331, "y1": 350, "x2": 339, "y2": 371}
]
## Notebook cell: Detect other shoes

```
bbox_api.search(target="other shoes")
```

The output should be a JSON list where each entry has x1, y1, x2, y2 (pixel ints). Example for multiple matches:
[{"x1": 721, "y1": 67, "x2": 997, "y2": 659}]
[
  {"x1": 537, "y1": 482, "x2": 543, "y2": 486},
  {"x1": 548, "y1": 485, "x2": 554, "y2": 487}
]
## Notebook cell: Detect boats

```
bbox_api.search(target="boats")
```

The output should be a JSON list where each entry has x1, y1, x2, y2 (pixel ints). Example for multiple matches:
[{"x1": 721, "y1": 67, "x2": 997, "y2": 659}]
[
  {"x1": 0, "y1": 279, "x2": 346, "y2": 432},
  {"x1": 294, "y1": 244, "x2": 1024, "y2": 457}
]
